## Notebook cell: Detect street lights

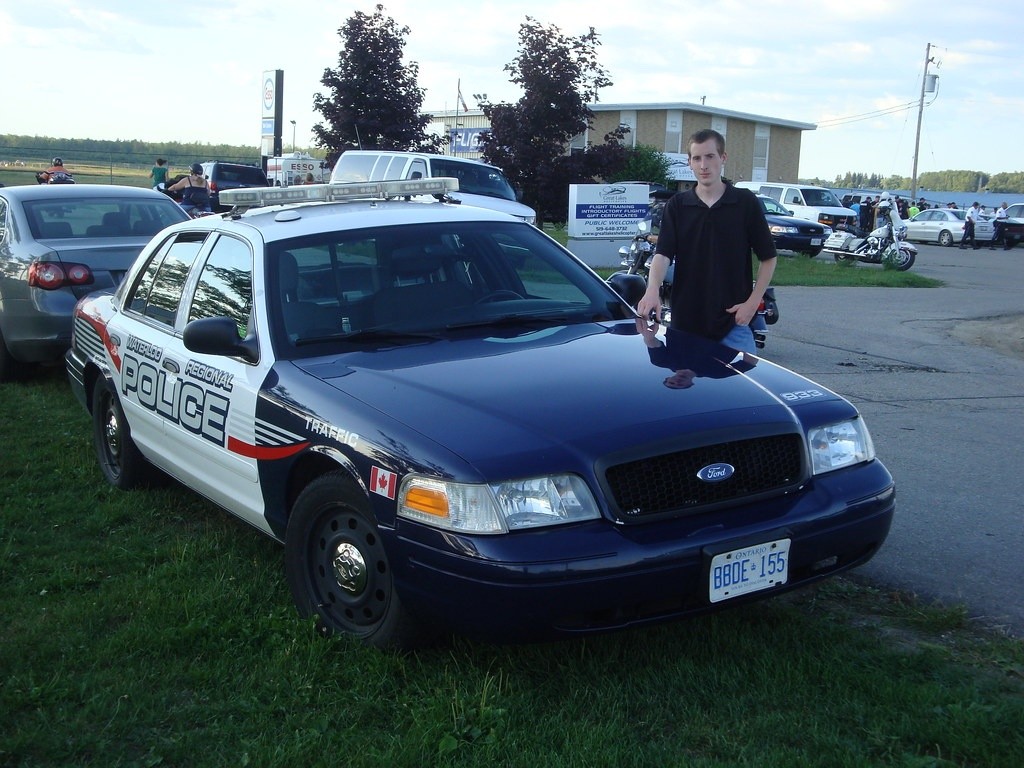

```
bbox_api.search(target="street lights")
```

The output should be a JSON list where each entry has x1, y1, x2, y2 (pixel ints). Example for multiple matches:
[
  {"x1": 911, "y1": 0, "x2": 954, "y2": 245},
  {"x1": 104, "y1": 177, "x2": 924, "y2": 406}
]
[{"x1": 290, "y1": 120, "x2": 296, "y2": 153}]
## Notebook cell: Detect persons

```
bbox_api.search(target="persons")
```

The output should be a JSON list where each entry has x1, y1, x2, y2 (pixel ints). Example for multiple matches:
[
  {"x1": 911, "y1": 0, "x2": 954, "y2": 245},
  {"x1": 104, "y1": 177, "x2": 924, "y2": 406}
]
[
  {"x1": 947, "y1": 202, "x2": 960, "y2": 218},
  {"x1": 636, "y1": 233, "x2": 674, "y2": 285},
  {"x1": 635, "y1": 317, "x2": 759, "y2": 390},
  {"x1": 635, "y1": 129, "x2": 777, "y2": 355},
  {"x1": 991, "y1": 208, "x2": 997, "y2": 214},
  {"x1": 168, "y1": 162, "x2": 211, "y2": 213},
  {"x1": 39, "y1": 157, "x2": 69, "y2": 185},
  {"x1": 305, "y1": 172, "x2": 315, "y2": 184},
  {"x1": 958, "y1": 202, "x2": 980, "y2": 249},
  {"x1": 842, "y1": 195, "x2": 880, "y2": 233},
  {"x1": 149, "y1": 159, "x2": 169, "y2": 191},
  {"x1": 990, "y1": 201, "x2": 1012, "y2": 251},
  {"x1": 979, "y1": 205, "x2": 987, "y2": 215},
  {"x1": 876, "y1": 191, "x2": 931, "y2": 229}
]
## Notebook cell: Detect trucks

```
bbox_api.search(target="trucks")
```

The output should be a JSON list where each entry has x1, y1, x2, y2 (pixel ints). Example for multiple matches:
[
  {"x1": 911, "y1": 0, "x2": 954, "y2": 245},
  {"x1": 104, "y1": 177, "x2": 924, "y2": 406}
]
[{"x1": 267, "y1": 152, "x2": 325, "y2": 185}]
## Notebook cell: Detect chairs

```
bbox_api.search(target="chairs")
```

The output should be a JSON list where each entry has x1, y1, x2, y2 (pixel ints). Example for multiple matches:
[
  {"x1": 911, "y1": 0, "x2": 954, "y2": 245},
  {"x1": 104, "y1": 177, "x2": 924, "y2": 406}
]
[
  {"x1": 42, "y1": 211, "x2": 133, "y2": 240},
  {"x1": 367, "y1": 243, "x2": 456, "y2": 326},
  {"x1": 249, "y1": 253, "x2": 331, "y2": 347}
]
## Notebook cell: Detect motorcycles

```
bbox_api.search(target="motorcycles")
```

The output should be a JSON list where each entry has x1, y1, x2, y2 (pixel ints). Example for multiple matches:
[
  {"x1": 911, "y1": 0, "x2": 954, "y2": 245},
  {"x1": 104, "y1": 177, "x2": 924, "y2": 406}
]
[
  {"x1": 821, "y1": 196, "x2": 919, "y2": 272},
  {"x1": 617, "y1": 202, "x2": 779, "y2": 349}
]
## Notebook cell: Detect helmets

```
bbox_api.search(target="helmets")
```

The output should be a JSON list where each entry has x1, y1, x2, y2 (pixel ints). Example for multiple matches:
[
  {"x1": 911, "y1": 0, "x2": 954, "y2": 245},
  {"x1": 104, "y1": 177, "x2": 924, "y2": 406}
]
[
  {"x1": 880, "y1": 192, "x2": 893, "y2": 201},
  {"x1": 878, "y1": 201, "x2": 893, "y2": 209},
  {"x1": 54, "y1": 158, "x2": 63, "y2": 166},
  {"x1": 189, "y1": 162, "x2": 203, "y2": 175}
]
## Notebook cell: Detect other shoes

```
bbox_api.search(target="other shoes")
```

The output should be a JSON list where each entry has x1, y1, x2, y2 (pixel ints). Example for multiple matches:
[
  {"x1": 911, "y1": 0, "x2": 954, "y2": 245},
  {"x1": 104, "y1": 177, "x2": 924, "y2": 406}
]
[
  {"x1": 989, "y1": 244, "x2": 997, "y2": 250},
  {"x1": 959, "y1": 243, "x2": 967, "y2": 249},
  {"x1": 972, "y1": 242, "x2": 980, "y2": 250},
  {"x1": 1004, "y1": 243, "x2": 1012, "y2": 250}
]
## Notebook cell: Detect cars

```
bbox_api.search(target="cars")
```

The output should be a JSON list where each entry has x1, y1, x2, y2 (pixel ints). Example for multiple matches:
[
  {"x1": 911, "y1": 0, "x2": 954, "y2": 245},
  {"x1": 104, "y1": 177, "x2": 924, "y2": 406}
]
[
  {"x1": 991, "y1": 203, "x2": 1024, "y2": 247},
  {"x1": 0, "y1": 183, "x2": 195, "y2": 374},
  {"x1": 62, "y1": 176, "x2": 899, "y2": 656},
  {"x1": 900, "y1": 207, "x2": 996, "y2": 248},
  {"x1": 754, "y1": 193, "x2": 833, "y2": 259}
]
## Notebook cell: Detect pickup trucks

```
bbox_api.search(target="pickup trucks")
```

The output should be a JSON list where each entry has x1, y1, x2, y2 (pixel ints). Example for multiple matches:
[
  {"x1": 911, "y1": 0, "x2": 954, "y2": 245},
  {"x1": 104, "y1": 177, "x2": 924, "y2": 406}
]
[{"x1": 734, "y1": 181, "x2": 858, "y2": 232}]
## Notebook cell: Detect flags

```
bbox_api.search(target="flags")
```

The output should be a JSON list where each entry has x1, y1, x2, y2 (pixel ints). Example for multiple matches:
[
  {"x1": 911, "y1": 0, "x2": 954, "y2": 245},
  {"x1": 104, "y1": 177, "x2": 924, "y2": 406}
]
[{"x1": 459, "y1": 91, "x2": 468, "y2": 112}]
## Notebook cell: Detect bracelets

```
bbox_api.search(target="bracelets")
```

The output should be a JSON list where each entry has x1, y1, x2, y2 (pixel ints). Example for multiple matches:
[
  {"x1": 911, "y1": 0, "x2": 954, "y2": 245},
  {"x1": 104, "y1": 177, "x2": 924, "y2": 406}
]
[{"x1": 649, "y1": 235, "x2": 652, "y2": 241}]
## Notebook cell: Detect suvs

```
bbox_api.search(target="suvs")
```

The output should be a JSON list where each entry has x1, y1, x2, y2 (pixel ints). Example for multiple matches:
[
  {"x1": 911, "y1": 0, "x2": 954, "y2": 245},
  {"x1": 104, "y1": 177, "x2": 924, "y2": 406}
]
[{"x1": 188, "y1": 159, "x2": 271, "y2": 212}]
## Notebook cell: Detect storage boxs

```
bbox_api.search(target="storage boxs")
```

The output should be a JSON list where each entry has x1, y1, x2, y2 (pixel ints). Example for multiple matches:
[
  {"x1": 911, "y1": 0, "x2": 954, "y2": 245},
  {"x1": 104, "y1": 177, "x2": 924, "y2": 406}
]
[
  {"x1": 47, "y1": 173, "x2": 75, "y2": 185},
  {"x1": 824, "y1": 232, "x2": 852, "y2": 251}
]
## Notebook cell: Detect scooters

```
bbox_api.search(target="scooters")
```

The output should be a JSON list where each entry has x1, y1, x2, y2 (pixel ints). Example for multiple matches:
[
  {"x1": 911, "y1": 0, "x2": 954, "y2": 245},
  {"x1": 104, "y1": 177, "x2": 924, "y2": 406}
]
[
  {"x1": 36, "y1": 171, "x2": 75, "y2": 185},
  {"x1": 163, "y1": 182, "x2": 216, "y2": 219}
]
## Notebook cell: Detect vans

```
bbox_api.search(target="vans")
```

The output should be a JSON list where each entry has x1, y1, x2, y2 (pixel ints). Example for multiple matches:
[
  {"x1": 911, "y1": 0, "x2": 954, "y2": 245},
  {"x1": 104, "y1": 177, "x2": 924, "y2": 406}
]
[{"x1": 328, "y1": 150, "x2": 537, "y2": 271}]
